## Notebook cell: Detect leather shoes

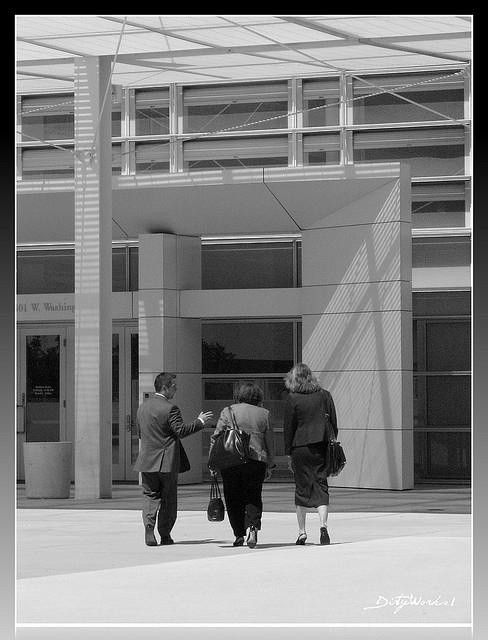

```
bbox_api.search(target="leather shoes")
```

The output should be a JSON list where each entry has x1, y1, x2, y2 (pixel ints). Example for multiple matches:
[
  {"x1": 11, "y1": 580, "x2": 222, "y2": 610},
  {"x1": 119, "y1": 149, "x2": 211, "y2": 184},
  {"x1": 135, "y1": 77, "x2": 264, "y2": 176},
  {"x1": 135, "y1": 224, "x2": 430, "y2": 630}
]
[
  {"x1": 145, "y1": 524, "x2": 157, "y2": 545},
  {"x1": 161, "y1": 537, "x2": 173, "y2": 544}
]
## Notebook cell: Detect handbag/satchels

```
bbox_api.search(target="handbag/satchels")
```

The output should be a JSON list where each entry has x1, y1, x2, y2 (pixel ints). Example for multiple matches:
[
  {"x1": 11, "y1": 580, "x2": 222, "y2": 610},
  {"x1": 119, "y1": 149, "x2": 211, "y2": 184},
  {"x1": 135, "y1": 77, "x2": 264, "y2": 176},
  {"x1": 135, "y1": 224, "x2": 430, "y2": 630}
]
[
  {"x1": 326, "y1": 440, "x2": 345, "y2": 477},
  {"x1": 208, "y1": 428, "x2": 251, "y2": 472},
  {"x1": 208, "y1": 498, "x2": 224, "y2": 521}
]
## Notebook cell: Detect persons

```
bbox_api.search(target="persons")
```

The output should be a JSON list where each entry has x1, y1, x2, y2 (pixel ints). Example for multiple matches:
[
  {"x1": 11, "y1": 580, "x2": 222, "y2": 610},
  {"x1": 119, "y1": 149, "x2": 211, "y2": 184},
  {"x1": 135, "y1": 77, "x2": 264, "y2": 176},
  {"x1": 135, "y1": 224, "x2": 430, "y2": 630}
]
[
  {"x1": 207, "y1": 382, "x2": 276, "y2": 548},
  {"x1": 132, "y1": 372, "x2": 214, "y2": 546},
  {"x1": 283, "y1": 364, "x2": 338, "y2": 545}
]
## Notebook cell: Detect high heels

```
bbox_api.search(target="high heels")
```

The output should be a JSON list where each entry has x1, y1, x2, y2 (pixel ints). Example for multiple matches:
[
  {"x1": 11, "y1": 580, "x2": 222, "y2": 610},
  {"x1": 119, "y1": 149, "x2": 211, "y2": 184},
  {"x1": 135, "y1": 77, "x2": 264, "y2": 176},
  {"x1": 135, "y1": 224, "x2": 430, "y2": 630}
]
[
  {"x1": 247, "y1": 526, "x2": 257, "y2": 543},
  {"x1": 320, "y1": 527, "x2": 330, "y2": 545},
  {"x1": 296, "y1": 533, "x2": 307, "y2": 544},
  {"x1": 233, "y1": 536, "x2": 244, "y2": 545}
]
